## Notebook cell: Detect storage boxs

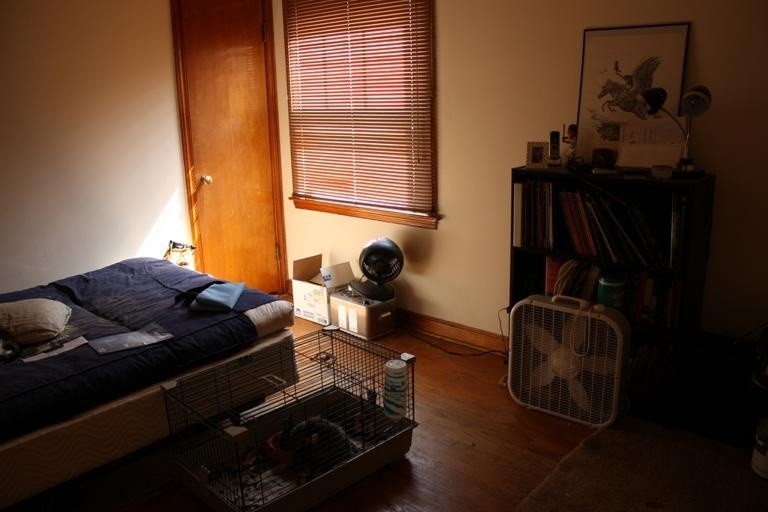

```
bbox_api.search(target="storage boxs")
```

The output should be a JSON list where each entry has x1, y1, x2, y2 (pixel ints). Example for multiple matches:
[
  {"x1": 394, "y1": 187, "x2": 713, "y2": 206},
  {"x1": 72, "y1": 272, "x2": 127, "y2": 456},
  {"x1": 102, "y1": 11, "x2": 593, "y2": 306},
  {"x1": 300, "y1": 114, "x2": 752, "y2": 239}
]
[
  {"x1": 292, "y1": 254, "x2": 356, "y2": 325},
  {"x1": 331, "y1": 292, "x2": 397, "y2": 340}
]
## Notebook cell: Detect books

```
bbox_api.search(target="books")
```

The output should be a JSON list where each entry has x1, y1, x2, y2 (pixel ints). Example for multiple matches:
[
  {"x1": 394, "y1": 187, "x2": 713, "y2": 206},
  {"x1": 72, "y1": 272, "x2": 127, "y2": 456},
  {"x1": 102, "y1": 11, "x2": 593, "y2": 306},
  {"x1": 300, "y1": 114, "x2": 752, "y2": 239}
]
[{"x1": 512, "y1": 181, "x2": 685, "y2": 333}]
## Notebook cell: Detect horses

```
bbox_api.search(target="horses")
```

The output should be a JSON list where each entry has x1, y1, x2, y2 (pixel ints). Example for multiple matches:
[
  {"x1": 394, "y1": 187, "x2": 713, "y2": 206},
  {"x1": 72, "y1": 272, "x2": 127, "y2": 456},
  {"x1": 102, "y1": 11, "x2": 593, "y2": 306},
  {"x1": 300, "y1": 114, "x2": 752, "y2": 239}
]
[{"x1": 597, "y1": 77, "x2": 665, "y2": 120}]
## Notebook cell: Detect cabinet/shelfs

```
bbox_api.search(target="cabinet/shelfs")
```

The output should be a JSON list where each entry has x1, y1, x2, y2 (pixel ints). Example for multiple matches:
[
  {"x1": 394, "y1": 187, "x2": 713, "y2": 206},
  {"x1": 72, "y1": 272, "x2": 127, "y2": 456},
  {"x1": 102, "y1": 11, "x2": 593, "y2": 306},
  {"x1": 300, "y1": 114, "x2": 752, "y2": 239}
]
[{"x1": 512, "y1": 164, "x2": 716, "y2": 419}]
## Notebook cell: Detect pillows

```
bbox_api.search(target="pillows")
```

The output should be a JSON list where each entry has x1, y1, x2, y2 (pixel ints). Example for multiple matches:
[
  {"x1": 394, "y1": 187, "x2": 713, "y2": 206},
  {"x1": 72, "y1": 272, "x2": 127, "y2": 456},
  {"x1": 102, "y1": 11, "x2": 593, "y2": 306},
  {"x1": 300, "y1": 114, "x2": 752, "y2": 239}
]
[{"x1": 0, "y1": 298, "x2": 72, "y2": 343}]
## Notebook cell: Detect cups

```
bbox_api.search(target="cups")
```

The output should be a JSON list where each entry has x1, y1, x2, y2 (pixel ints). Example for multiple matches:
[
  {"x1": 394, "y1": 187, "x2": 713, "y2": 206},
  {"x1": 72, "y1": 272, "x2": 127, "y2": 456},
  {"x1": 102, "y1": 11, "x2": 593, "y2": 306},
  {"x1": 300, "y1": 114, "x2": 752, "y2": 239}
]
[{"x1": 596, "y1": 273, "x2": 627, "y2": 308}]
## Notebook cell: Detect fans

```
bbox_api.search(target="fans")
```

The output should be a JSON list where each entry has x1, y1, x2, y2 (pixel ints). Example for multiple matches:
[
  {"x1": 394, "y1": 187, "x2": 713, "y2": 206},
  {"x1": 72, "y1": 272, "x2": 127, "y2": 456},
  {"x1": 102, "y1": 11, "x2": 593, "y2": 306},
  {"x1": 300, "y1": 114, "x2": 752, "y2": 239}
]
[
  {"x1": 348, "y1": 236, "x2": 405, "y2": 301},
  {"x1": 507, "y1": 294, "x2": 630, "y2": 431}
]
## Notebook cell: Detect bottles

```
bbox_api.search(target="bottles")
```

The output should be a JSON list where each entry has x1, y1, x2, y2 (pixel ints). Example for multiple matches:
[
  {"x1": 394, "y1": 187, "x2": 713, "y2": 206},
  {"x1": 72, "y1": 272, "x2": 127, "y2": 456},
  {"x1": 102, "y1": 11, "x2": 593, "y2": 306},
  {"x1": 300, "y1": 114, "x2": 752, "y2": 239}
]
[{"x1": 383, "y1": 358, "x2": 410, "y2": 423}]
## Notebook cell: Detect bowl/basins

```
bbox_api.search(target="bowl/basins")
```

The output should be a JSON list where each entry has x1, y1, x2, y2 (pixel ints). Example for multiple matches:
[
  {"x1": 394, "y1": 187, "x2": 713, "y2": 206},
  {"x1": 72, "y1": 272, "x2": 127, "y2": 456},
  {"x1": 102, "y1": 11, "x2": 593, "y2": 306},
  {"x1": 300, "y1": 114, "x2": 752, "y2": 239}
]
[{"x1": 650, "y1": 165, "x2": 673, "y2": 179}]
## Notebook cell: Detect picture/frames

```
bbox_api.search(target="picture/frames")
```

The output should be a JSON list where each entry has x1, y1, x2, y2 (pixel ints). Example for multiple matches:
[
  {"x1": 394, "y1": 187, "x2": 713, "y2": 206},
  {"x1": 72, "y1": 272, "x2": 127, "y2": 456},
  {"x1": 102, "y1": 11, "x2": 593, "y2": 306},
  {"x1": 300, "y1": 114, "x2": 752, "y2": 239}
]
[
  {"x1": 575, "y1": 21, "x2": 691, "y2": 166},
  {"x1": 527, "y1": 141, "x2": 550, "y2": 169}
]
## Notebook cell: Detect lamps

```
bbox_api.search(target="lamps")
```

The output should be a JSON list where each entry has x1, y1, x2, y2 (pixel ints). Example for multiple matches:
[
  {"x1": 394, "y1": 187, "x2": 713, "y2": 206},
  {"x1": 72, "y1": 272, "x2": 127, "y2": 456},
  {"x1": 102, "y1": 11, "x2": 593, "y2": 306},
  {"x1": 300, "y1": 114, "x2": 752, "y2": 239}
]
[{"x1": 641, "y1": 86, "x2": 711, "y2": 171}]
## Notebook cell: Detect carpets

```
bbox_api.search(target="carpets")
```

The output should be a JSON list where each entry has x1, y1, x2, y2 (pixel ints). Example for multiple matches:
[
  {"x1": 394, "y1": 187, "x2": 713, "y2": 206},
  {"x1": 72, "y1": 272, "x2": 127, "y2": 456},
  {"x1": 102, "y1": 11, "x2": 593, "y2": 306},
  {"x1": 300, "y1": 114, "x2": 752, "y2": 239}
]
[{"x1": 512, "y1": 426, "x2": 768, "y2": 512}]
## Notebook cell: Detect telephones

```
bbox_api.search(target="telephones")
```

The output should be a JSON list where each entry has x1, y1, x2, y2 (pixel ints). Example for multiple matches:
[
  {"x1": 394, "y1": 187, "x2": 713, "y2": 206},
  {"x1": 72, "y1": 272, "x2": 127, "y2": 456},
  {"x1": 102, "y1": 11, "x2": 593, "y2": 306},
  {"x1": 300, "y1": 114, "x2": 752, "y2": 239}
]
[{"x1": 547, "y1": 130, "x2": 562, "y2": 165}]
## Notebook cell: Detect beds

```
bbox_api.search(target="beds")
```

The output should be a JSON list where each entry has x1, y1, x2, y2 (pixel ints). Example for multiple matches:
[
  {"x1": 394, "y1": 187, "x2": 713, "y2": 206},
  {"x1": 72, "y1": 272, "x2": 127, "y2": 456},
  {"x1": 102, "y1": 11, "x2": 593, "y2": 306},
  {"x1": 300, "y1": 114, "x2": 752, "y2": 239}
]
[{"x1": 0, "y1": 257, "x2": 298, "y2": 510}]
